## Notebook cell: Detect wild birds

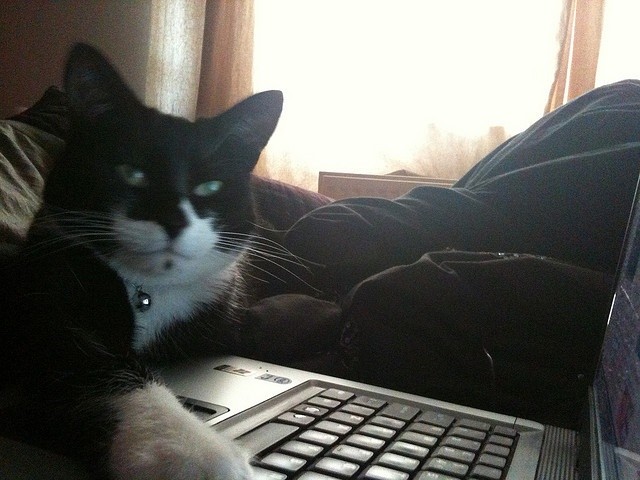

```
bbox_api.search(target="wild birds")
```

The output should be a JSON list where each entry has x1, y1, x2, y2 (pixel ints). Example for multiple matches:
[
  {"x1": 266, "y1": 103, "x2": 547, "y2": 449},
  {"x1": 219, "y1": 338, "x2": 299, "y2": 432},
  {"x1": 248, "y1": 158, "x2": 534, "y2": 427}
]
[{"x1": 144, "y1": 175, "x2": 640, "y2": 480}]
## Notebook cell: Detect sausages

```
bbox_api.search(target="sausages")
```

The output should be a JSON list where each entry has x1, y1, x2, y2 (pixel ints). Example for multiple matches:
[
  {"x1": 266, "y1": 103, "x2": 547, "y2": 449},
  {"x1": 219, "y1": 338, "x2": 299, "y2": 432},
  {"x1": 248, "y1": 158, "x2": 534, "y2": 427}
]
[{"x1": 0, "y1": 41, "x2": 328, "y2": 480}]
